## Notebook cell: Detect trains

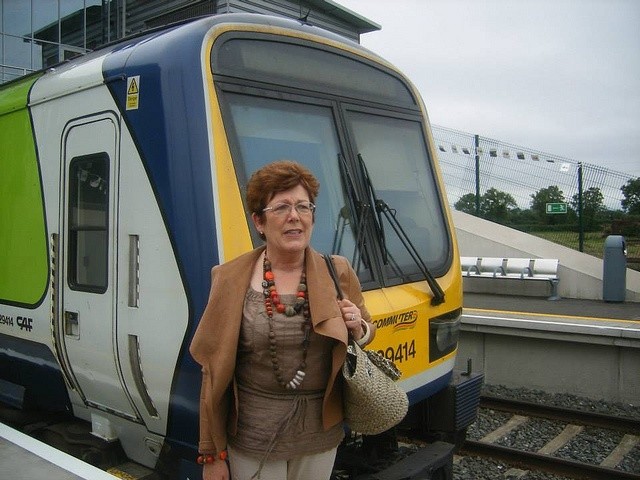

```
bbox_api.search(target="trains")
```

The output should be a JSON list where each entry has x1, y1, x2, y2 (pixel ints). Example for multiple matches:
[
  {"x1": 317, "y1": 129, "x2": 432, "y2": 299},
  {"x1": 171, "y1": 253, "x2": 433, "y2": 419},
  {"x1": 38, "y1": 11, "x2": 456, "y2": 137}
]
[{"x1": 2, "y1": 13, "x2": 485, "y2": 480}]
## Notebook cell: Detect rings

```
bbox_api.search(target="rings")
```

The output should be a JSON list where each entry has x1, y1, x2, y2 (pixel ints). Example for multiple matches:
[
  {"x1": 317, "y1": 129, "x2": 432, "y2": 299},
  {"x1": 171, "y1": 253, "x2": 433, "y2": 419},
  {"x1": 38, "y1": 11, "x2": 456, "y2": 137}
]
[{"x1": 350, "y1": 314, "x2": 356, "y2": 320}]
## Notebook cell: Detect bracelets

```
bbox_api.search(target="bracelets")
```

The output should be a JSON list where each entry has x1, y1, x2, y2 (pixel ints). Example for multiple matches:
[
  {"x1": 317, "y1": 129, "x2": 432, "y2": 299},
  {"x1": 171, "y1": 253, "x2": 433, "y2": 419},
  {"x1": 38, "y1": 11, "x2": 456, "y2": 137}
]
[{"x1": 197, "y1": 451, "x2": 228, "y2": 463}]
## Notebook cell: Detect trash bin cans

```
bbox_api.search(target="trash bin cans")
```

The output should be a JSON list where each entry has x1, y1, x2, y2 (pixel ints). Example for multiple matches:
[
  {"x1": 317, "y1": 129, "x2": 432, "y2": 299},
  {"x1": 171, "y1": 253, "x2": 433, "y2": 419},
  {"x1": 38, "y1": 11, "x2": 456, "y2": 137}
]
[{"x1": 601, "y1": 235, "x2": 627, "y2": 304}]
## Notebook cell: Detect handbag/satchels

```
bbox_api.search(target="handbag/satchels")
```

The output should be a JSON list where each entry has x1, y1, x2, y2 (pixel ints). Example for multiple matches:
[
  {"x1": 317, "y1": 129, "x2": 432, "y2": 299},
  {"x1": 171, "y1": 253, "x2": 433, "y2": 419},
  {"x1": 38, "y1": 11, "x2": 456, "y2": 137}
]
[{"x1": 323, "y1": 253, "x2": 409, "y2": 434}]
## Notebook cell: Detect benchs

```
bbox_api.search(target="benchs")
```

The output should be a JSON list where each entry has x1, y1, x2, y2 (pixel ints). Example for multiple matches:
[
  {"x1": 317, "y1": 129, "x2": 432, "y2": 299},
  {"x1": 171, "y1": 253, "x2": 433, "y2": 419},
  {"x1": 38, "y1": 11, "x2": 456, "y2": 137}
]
[{"x1": 460, "y1": 255, "x2": 561, "y2": 301}]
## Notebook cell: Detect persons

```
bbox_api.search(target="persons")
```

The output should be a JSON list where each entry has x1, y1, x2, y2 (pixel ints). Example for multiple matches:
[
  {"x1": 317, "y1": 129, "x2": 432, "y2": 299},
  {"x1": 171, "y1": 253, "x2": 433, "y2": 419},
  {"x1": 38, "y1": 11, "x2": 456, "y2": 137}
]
[{"x1": 189, "y1": 161, "x2": 377, "y2": 479}]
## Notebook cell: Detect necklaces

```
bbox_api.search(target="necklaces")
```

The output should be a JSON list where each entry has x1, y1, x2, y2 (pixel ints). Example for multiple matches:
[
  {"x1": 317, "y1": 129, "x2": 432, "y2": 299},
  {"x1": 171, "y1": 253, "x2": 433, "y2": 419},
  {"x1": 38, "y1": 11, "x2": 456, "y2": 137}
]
[{"x1": 262, "y1": 247, "x2": 312, "y2": 397}]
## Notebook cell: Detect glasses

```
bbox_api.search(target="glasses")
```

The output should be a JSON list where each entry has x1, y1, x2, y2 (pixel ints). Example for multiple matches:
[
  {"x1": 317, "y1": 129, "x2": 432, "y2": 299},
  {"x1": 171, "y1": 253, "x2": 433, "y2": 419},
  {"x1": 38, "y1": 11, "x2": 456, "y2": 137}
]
[{"x1": 260, "y1": 201, "x2": 315, "y2": 217}]
